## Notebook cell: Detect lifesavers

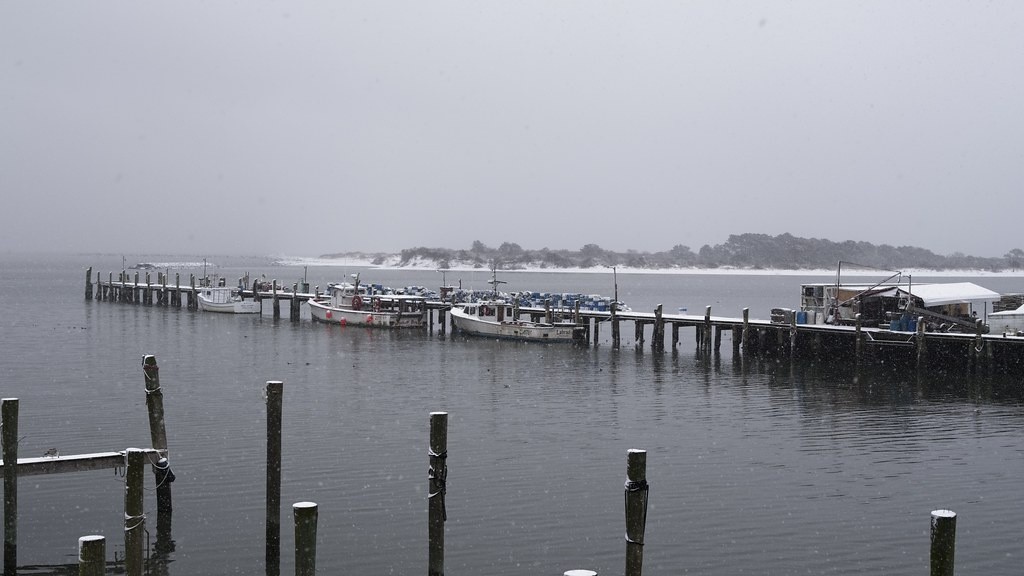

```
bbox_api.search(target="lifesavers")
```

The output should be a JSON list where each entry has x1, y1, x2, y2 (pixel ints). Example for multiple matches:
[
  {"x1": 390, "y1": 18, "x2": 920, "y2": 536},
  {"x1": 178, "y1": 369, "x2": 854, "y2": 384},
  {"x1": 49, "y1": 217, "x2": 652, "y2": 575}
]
[
  {"x1": 481, "y1": 305, "x2": 490, "y2": 315},
  {"x1": 351, "y1": 295, "x2": 362, "y2": 309}
]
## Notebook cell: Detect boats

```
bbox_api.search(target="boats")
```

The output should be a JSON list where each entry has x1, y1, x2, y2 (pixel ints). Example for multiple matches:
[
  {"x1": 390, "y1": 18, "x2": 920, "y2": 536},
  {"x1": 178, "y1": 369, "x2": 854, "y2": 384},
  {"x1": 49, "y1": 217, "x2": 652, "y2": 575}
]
[
  {"x1": 204, "y1": 287, "x2": 262, "y2": 315},
  {"x1": 308, "y1": 293, "x2": 427, "y2": 328},
  {"x1": 449, "y1": 302, "x2": 589, "y2": 344}
]
